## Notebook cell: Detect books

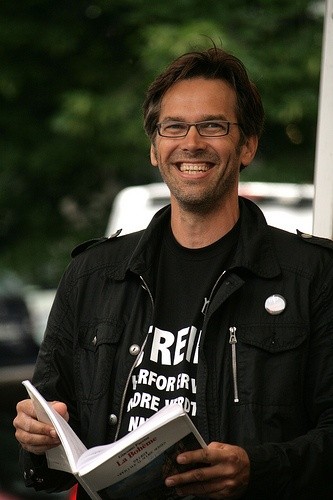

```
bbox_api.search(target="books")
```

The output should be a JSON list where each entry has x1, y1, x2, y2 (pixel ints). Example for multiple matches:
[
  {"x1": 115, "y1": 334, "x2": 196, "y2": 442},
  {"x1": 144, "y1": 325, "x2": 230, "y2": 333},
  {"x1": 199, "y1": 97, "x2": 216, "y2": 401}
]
[{"x1": 19, "y1": 380, "x2": 209, "y2": 500}]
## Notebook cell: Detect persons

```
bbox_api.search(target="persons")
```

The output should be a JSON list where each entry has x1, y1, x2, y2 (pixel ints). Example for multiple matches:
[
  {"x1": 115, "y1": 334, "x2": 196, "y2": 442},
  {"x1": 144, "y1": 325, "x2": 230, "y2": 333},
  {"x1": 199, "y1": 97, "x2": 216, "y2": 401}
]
[{"x1": 12, "y1": 33, "x2": 333, "y2": 500}]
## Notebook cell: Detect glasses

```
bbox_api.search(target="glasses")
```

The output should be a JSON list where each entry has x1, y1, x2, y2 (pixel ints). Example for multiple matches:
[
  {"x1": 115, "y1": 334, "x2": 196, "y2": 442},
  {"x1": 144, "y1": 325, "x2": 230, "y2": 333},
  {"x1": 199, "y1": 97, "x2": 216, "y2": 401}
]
[{"x1": 156, "y1": 120, "x2": 239, "y2": 137}]
